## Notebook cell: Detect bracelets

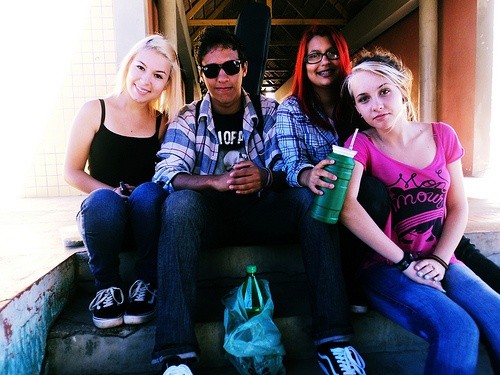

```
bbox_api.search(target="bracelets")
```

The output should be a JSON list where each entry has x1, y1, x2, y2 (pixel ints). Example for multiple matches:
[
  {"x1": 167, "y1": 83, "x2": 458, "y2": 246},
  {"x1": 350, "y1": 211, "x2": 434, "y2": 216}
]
[
  {"x1": 428, "y1": 255, "x2": 449, "y2": 270},
  {"x1": 396, "y1": 252, "x2": 412, "y2": 271}
]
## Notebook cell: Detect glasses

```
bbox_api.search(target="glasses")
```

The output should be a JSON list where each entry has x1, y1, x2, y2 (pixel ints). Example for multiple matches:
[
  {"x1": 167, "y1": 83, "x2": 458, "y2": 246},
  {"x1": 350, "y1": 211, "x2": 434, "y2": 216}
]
[
  {"x1": 200, "y1": 59, "x2": 242, "y2": 78},
  {"x1": 304, "y1": 48, "x2": 340, "y2": 64}
]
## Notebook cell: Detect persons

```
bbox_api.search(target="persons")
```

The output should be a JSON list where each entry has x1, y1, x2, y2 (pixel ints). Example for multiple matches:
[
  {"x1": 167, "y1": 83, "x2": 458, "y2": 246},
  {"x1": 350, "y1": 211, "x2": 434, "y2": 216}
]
[
  {"x1": 63, "y1": 35, "x2": 181, "y2": 328},
  {"x1": 276, "y1": 28, "x2": 500, "y2": 375},
  {"x1": 340, "y1": 48, "x2": 500, "y2": 375},
  {"x1": 152, "y1": 26, "x2": 365, "y2": 375}
]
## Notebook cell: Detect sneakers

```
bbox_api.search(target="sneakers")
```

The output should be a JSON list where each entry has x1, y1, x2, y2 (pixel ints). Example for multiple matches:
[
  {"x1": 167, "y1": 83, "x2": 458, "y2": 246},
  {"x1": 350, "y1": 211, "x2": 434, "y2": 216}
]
[
  {"x1": 124, "y1": 279, "x2": 158, "y2": 324},
  {"x1": 315, "y1": 343, "x2": 366, "y2": 375},
  {"x1": 160, "y1": 356, "x2": 198, "y2": 375},
  {"x1": 88, "y1": 286, "x2": 124, "y2": 329}
]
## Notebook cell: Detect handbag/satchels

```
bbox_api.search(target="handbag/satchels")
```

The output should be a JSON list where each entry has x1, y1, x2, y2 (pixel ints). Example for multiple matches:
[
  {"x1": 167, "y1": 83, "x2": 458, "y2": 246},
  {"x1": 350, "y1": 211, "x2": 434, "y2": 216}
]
[{"x1": 221, "y1": 279, "x2": 285, "y2": 375}]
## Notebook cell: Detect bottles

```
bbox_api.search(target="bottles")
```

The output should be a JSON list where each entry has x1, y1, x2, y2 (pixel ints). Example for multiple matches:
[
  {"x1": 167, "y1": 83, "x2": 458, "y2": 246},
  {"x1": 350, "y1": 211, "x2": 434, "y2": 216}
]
[
  {"x1": 310, "y1": 145, "x2": 358, "y2": 224},
  {"x1": 241, "y1": 264, "x2": 264, "y2": 319}
]
